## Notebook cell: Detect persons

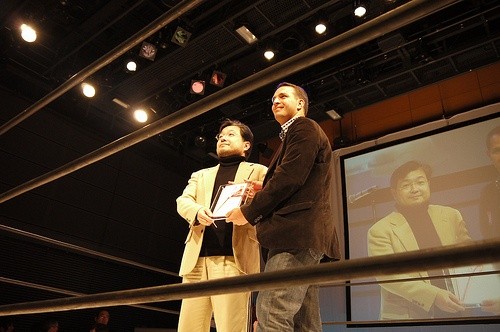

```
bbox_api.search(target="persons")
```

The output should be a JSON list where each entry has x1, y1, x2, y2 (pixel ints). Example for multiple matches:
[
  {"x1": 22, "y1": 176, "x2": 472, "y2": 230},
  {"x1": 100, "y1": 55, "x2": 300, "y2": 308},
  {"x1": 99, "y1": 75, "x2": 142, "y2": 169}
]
[
  {"x1": 366, "y1": 125, "x2": 500, "y2": 318},
  {"x1": 226, "y1": 82, "x2": 341, "y2": 332},
  {"x1": 176, "y1": 120, "x2": 270, "y2": 332}
]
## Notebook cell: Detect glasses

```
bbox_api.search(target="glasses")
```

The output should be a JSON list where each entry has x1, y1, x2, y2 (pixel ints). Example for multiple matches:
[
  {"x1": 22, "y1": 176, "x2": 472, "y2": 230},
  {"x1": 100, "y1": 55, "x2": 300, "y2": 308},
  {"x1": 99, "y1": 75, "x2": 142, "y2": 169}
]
[
  {"x1": 215, "y1": 132, "x2": 241, "y2": 140},
  {"x1": 272, "y1": 93, "x2": 298, "y2": 103}
]
